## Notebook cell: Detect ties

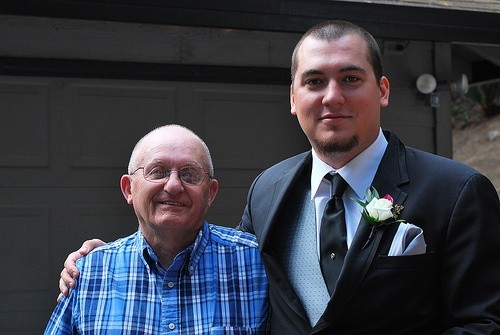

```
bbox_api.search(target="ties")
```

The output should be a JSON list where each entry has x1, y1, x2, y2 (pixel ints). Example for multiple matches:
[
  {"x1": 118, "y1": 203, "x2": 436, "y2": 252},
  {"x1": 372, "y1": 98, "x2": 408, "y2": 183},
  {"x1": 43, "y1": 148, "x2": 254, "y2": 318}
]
[{"x1": 319, "y1": 172, "x2": 349, "y2": 298}]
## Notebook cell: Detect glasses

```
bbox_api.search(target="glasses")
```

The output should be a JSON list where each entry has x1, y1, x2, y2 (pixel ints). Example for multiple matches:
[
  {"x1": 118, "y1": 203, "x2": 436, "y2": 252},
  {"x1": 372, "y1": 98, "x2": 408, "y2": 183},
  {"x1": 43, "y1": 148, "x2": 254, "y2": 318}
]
[{"x1": 130, "y1": 160, "x2": 213, "y2": 185}]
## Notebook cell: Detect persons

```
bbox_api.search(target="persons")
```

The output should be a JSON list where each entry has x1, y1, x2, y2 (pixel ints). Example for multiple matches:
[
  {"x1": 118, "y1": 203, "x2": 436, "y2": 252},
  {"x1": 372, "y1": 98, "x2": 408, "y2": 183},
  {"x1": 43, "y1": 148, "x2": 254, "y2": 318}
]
[
  {"x1": 45, "y1": 124, "x2": 271, "y2": 335},
  {"x1": 56, "y1": 21, "x2": 500, "y2": 335}
]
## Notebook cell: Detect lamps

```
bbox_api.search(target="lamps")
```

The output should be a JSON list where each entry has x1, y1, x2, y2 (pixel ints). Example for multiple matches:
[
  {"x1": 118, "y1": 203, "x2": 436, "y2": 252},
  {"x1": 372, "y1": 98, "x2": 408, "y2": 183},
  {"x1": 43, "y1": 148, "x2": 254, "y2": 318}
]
[{"x1": 415, "y1": 74, "x2": 469, "y2": 108}]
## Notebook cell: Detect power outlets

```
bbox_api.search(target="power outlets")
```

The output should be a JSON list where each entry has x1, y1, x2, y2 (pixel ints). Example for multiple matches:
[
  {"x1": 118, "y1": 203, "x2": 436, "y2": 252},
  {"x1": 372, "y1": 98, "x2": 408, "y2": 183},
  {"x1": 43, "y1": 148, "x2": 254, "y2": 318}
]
[{"x1": 383, "y1": 41, "x2": 406, "y2": 57}]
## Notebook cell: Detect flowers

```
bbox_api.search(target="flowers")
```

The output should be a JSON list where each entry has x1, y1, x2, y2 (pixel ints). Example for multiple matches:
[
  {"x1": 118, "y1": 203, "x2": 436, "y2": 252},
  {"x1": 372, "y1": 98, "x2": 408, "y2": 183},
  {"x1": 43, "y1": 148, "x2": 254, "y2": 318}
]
[{"x1": 350, "y1": 185, "x2": 407, "y2": 249}]
